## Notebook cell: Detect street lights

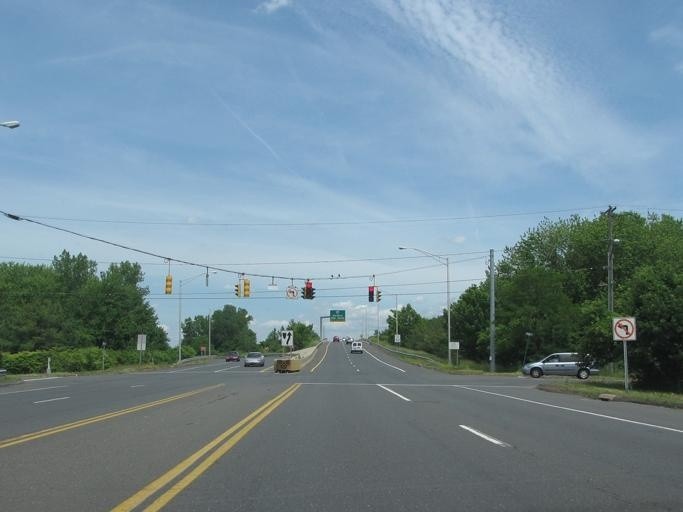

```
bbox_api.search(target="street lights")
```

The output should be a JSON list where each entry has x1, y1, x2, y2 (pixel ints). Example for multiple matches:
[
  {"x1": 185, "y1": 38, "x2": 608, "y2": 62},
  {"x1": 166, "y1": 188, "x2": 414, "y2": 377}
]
[
  {"x1": 607, "y1": 238, "x2": 620, "y2": 313},
  {"x1": 0, "y1": 121, "x2": 19, "y2": 129},
  {"x1": 397, "y1": 246, "x2": 451, "y2": 367}
]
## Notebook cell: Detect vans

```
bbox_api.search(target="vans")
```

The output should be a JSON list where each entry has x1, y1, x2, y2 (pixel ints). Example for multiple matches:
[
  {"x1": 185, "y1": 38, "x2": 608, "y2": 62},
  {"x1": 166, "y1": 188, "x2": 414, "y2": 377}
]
[{"x1": 520, "y1": 352, "x2": 599, "y2": 378}]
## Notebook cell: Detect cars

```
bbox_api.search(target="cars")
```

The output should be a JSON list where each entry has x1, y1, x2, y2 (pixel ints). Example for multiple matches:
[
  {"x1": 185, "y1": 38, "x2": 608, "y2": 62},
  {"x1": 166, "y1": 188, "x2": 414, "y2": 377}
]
[
  {"x1": 244, "y1": 351, "x2": 264, "y2": 367},
  {"x1": 333, "y1": 335, "x2": 362, "y2": 353},
  {"x1": 224, "y1": 351, "x2": 240, "y2": 362}
]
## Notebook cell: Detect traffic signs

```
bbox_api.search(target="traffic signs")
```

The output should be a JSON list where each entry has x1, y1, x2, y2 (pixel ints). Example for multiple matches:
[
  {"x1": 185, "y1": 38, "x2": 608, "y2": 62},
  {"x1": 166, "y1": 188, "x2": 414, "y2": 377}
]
[{"x1": 329, "y1": 309, "x2": 344, "y2": 322}]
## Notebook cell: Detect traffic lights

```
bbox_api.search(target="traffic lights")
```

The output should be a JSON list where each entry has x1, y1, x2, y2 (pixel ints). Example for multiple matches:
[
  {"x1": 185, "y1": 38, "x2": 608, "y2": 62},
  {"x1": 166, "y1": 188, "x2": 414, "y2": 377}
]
[
  {"x1": 301, "y1": 287, "x2": 306, "y2": 299},
  {"x1": 376, "y1": 291, "x2": 381, "y2": 302},
  {"x1": 234, "y1": 283, "x2": 241, "y2": 297},
  {"x1": 309, "y1": 288, "x2": 315, "y2": 300},
  {"x1": 306, "y1": 282, "x2": 312, "y2": 289},
  {"x1": 368, "y1": 286, "x2": 374, "y2": 302}
]
